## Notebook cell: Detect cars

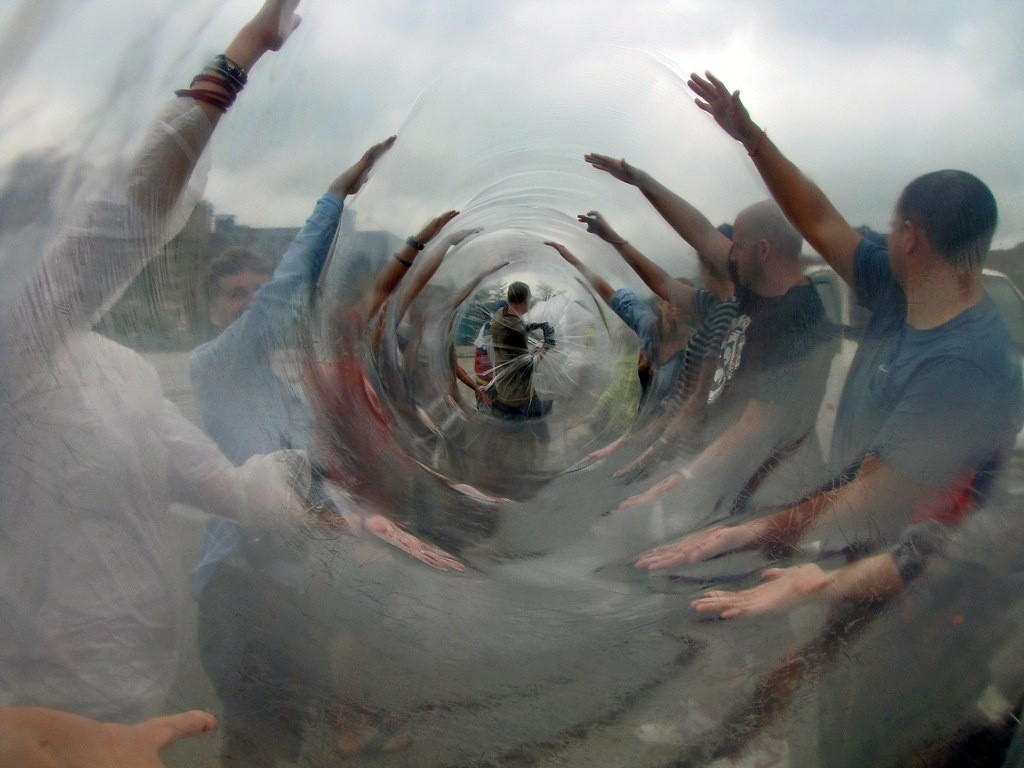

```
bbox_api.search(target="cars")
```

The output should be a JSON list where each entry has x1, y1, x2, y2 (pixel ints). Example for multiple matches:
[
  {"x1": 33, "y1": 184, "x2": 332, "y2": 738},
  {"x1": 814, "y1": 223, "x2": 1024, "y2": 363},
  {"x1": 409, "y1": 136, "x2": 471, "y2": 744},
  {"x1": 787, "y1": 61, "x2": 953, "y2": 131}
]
[{"x1": 800, "y1": 263, "x2": 1024, "y2": 478}]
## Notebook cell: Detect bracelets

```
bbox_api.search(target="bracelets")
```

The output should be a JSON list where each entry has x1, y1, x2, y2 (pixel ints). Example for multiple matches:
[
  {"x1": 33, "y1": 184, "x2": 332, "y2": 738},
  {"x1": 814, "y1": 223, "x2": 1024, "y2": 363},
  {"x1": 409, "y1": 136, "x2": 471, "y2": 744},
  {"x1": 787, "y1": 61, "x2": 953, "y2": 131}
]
[
  {"x1": 211, "y1": 55, "x2": 248, "y2": 85},
  {"x1": 657, "y1": 436, "x2": 668, "y2": 447},
  {"x1": 175, "y1": 89, "x2": 228, "y2": 113},
  {"x1": 405, "y1": 236, "x2": 424, "y2": 251},
  {"x1": 679, "y1": 470, "x2": 694, "y2": 487},
  {"x1": 189, "y1": 73, "x2": 237, "y2": 101},
  {"x1": 177, "y1": 93, "x2": 230, "y2": 109},
  {"x1": 748, "y1": 132, "x2": 766, "y2": 157},
  {"x1": 202, "y1": 65, "x2": 244, "y2": 91},
  {"x1": 394, "y1": 252, "x2": 412, "y2": 268}
]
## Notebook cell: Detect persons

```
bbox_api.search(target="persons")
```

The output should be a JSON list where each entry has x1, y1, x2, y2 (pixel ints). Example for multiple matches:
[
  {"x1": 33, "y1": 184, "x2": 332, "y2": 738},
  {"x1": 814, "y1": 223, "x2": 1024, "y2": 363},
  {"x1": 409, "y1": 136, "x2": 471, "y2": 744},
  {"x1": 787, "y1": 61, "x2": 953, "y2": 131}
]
[{"x1": 0, "y1": 0, "x2": 1024, "y2": 768}]
